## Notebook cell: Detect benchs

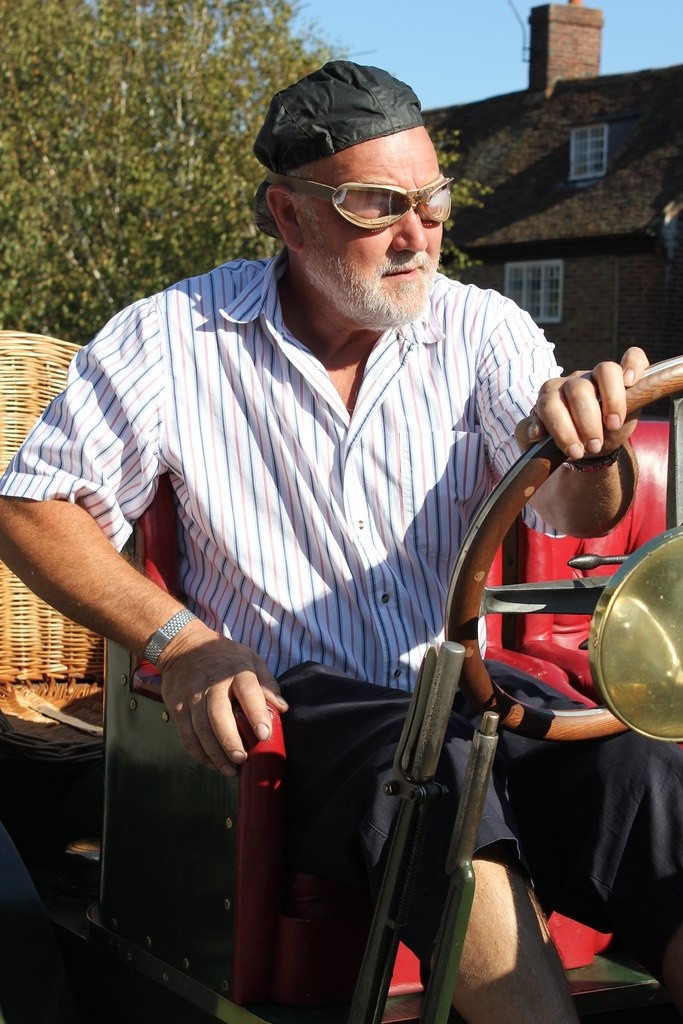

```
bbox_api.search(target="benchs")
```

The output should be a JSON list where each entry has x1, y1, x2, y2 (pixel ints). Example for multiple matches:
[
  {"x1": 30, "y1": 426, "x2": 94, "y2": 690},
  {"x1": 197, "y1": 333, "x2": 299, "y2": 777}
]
[{"x1": 1, "y1": 325, "x2": 670, "y2": 1014}]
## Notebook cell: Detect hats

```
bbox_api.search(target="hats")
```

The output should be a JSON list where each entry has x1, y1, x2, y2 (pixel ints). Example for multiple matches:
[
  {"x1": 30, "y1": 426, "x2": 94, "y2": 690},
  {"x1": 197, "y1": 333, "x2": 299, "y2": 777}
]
[{"x1": 251, "y1": 60, "x2": 428, "y2": 240}]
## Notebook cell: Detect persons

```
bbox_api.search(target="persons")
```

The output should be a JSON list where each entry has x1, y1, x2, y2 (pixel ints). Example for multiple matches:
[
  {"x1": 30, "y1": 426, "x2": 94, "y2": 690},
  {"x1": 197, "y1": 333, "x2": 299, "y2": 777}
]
[{"x1": 2, "y1": 58, "x2": 650, "y2": 1024}]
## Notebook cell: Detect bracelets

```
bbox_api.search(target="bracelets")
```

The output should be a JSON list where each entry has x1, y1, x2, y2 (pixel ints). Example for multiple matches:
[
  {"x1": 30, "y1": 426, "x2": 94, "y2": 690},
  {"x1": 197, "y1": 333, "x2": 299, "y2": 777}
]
[
  {"x1": 557, "y1": 444, "x2": 623, "y2": 474},
  {"x1": 143, "y1": 607, "x2": 199, "y2": 666}
]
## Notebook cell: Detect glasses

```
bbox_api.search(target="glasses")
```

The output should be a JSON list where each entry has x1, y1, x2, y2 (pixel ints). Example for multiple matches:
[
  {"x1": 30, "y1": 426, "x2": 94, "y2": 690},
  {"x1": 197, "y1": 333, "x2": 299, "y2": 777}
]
[{"x1": 266, "y1": 168, "x2": 455, "y2": 231}]
